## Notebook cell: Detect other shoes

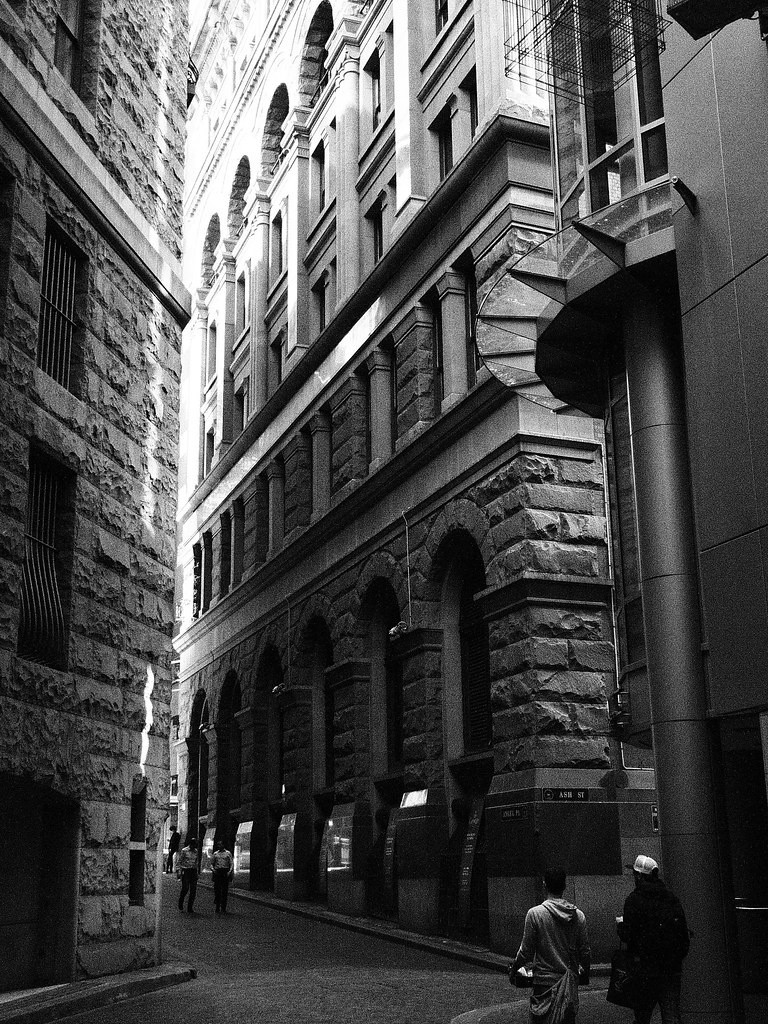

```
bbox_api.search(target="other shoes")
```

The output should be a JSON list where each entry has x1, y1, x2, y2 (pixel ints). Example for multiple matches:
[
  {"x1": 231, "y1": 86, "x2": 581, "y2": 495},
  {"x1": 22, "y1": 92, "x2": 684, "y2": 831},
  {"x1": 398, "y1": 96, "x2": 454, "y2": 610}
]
[
  {"x1": 179, "y1": 907, "x2": 184, "y2": 910},
  {"x1": 187, "y1": 910, "x2": 194, "y2": 913},
  {"x1": 215, "y1": 909, "x2": 227, "y2": 914}
]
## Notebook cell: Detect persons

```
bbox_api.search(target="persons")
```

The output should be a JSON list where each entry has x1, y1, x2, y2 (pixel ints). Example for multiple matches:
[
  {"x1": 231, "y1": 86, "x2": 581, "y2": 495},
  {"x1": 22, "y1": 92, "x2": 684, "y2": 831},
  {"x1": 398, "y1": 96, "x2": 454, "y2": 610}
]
[
  {"x1": 210, "y1": 840, "x2": 233, "y2": 915},
  {"x1": 508, "y1": 867, "x2": 591, "y2": 1024},
  {"x1": 178, "y1": 838, "x2": 199, "y2": 914},
  {"x1": 616, "y1": 855, "x2": 690, "y2": 1024},
  {"x1": 166, "y1": 826, "x2": 181, "y2": 874}
]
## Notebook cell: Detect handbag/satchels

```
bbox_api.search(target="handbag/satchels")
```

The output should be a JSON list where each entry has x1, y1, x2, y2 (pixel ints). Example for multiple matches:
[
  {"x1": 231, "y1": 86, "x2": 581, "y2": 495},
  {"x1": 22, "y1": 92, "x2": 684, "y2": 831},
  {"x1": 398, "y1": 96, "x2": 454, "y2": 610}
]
[
  {"x1": 529, "y1": 971, "x2": 578, "y2": 1024},
  {"x1": 606, "y1": 940, "x2": 662, "y2": 1014}
]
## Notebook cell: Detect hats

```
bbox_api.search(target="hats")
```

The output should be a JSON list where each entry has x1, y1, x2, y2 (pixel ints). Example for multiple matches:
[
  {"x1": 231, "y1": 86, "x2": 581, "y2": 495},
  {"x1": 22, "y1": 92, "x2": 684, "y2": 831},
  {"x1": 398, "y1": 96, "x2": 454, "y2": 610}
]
[{"x1": 626, "y1": 855, "x2": 659, "y2": 875}]
